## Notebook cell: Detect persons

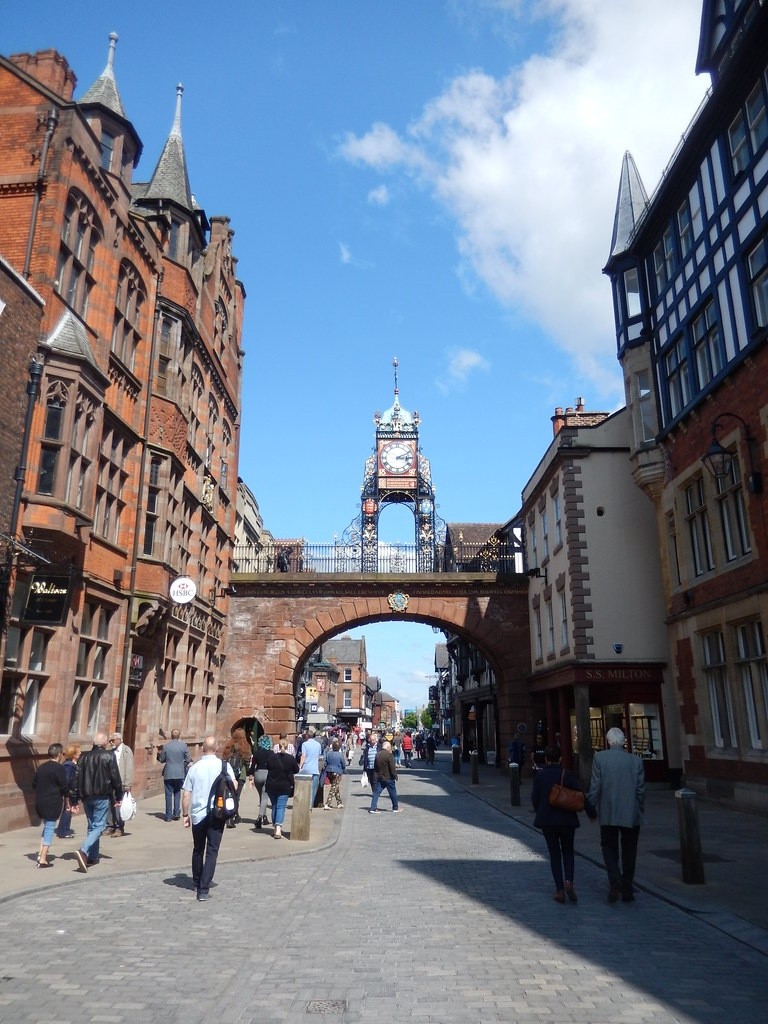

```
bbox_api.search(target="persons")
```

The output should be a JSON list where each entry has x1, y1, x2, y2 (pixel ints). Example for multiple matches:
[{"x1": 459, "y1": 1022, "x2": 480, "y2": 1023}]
[
  {"x1": 510, "y1": 733, "x2": 561, "y2": 789},
  {"x1": 182, "y1": 736, "x2": 238, "y2": 901},
  {"x1": 589, "y1": 728, "x2": 647, "y2": 902},
  {"x1": 532, "y1": 744, "x2": 597, "y2": 902},
  {"x1": 33, "y1": 726, "x2": 461, "y2": 874},
  {"x1": 277, "y1": 546, "x2": 295, "y2": 574}
]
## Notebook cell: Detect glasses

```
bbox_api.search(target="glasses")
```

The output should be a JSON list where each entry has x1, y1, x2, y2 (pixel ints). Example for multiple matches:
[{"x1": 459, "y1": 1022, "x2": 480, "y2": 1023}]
[{"x1": 109, "y1": 740, "x2": 114, "y2": 743}]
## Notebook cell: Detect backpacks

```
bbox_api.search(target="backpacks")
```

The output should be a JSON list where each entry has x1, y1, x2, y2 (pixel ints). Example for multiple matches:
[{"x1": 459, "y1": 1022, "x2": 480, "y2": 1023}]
[
  {"x1": 207, "y1": 760, "x2": 238, "y2": 821},
  {"x1": 229, "y1": 746, "x2": 243, "y2": 781}
]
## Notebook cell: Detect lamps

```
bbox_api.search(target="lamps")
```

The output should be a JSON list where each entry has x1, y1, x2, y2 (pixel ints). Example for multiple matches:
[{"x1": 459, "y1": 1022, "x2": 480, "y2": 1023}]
[{"x1": 700, "y1": 412, "x2": 763, "y2": 494}]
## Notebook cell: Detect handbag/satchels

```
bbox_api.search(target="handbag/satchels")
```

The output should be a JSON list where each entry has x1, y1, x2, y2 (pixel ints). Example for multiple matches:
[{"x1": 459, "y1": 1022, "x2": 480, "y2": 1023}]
[
  {"x1": 361, "y1": 771, "x2": 368, "y2": 788},
  {"x1": 288, "y1": 783, "x2": 294, "y2": 797},
  {"x1": 549, "y1": 767, "x2": 584, "y2": 812},
  {"x1": 120, "y1": 791, "x2": 136, "y2": 821}
]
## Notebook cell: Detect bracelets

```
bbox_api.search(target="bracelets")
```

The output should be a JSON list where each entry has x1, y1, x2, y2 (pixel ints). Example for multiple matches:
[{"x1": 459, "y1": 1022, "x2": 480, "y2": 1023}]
[{"x1": 183, "y1": 814, "x2": 188, "y2": 817}]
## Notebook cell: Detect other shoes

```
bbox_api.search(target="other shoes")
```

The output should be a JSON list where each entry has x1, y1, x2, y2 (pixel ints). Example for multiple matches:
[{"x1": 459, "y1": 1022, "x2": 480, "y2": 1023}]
[
  {"x1": 58, "y1": 829, "x2": 75, "y2": 838},
  {"x1": 75, "y1": 849, "x2": 99, "y2": 872},
  {"x1": 192, "y1": 886, "x2": 208, "y2": 900},
  {"x1": 337, "y1": 804, "x2": 343, "y2": 808},
  {"x1": 565, "y1": 883, "x2": 577, "y2": 901},
  {"x1": 254, "y1": 815, "x2": 270, "y2": 829},
  {"x1": 424, "y1": 761, "x2": 434, "y2": 766},
  {"x1": 609, "y1": 883, "x2": 633, "y2": 903},
  {"x1": 102, "y1": 830, "x2": 124, "y2": 837},
  {"x1": 553, "y1": 892, "x2": 565, "y2": 903},
  {"x1": 393, "y1": 809, "x2": 404, "y2": 813},
  {"x1": 227, "y1": 817, "x2": 244, "y2": 828},
  {"x1": 370, "y1": 809, "x2": 381, "y2": 814},
  {"x1": 37, "y1": 855, "x2": 54, "y2": 867},
  {"x1": 164, "y1": 816, "x2": 180, "y2": 821},
  {"x1": 272, "y1": 829, "x2": 282, "y2": 839},
  {"x1": 324, "y1": 806, "x2": 332, "y2": 809},
  {"x1": 405, "y1": 763, "x2": 414, "y2": 768},
  {"x1": 395, "y1": 764, "x2": 401, "y2": 768}
]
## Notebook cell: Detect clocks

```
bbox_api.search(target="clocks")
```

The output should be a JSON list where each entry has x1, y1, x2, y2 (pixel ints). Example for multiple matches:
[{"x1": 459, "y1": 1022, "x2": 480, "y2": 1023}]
[{"x1": 380, "y1": 441, "x2": 414, "y2": 475}]
